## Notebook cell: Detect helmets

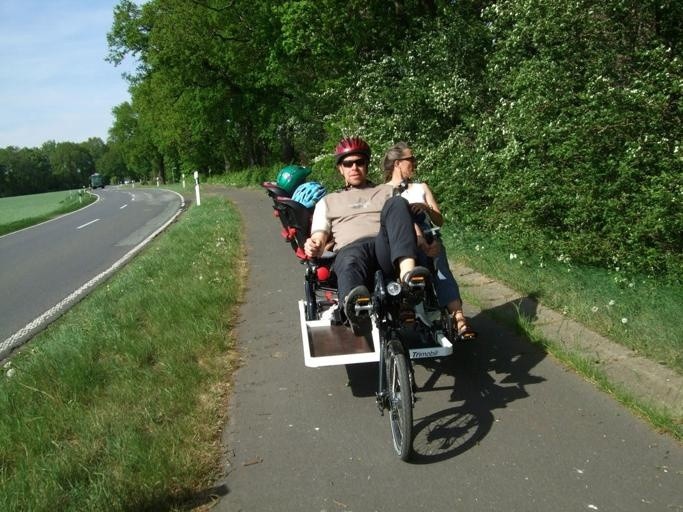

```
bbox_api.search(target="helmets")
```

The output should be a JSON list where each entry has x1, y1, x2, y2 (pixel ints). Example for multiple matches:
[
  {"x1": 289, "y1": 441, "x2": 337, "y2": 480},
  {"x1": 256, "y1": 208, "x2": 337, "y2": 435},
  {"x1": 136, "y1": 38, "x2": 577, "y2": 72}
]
[
  {"x1": 277, "y1": 164, "x2": 313, "y2": 194},
  {"x1": 292, "y1": 180, "x2": 328, "y2": 209},
  {"x1": 333, "y1": 136, "x2": 372, "y2": 167}
]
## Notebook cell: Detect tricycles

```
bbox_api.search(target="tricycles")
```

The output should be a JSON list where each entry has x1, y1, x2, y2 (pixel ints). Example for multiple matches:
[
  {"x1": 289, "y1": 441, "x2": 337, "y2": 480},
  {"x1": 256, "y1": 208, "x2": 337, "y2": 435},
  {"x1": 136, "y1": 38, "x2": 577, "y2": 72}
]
[{"x1": 303, "y1": 203, "x2": 469, "y2": 464}]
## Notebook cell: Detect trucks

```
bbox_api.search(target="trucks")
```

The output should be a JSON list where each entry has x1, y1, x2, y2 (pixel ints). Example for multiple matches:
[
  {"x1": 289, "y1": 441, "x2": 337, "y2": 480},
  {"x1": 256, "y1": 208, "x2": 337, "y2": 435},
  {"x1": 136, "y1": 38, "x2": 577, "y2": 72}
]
[{"x1": 90, "y1": 173, "x2": 105, "y2": 189}]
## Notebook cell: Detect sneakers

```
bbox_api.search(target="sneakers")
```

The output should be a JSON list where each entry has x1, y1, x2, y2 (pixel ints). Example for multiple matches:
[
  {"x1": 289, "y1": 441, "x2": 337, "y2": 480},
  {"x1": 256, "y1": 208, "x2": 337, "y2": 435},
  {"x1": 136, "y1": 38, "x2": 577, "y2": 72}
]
[
  {"x1": 447, "y1": 307, "x2": 479, "y2": 342},
  {"x1": 402, "y1": 266, "x2": 432, "y2": 307},
  {"x1": 344, "y1": 283, "x2": 374, "y2": 339},
  {"x1": 397, "y1": 309, "x2": 417, "y2": 324}
]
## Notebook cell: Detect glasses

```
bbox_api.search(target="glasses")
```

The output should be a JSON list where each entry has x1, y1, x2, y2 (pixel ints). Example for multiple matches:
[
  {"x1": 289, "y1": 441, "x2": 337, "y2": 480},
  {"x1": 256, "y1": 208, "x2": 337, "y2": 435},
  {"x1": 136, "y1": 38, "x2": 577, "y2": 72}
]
[
  {"x1": 340, "y1": 157, "x2": 368, "y2": 169},
  {"x1": 395, "y1": 156, "x2": 417, "y2": 163}
]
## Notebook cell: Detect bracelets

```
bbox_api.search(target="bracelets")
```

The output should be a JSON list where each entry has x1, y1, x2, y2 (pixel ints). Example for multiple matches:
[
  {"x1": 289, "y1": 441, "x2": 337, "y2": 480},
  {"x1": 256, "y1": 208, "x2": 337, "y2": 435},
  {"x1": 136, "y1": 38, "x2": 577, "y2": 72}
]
[{"x1": 429, "y1": 204, "x2": 433, "y2": 216}]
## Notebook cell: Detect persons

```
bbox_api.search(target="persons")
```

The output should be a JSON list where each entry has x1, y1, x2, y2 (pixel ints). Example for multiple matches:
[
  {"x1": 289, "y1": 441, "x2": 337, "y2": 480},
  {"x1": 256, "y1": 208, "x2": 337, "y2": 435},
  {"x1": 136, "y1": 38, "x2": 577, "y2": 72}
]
[
  {"x1": 384, "y1": 142, "x2": 478, "y2": 341},
  {"x1": 304, "y1": 136, "x2": 440, "y2": 336}
]
